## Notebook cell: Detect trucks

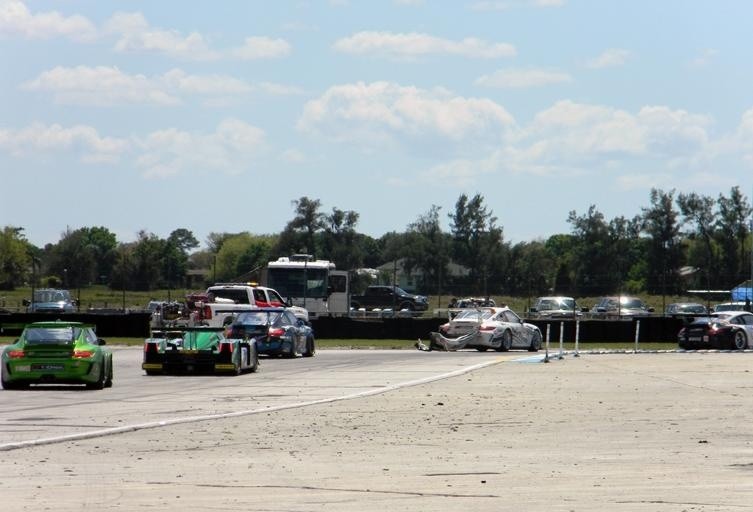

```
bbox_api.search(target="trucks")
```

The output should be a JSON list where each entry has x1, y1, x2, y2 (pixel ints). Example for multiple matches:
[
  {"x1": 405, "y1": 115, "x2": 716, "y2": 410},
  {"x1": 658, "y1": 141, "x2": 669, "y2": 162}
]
[{"x1": 266, "y1": 253, "x2": 349, "y2": 322}]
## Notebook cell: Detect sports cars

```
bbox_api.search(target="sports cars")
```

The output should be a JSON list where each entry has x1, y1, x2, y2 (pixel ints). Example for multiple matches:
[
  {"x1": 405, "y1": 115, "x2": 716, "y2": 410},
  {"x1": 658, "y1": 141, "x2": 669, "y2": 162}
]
[
  {"x1": 438, "y1": 298, "x2": 543, "y2": 352},
  {"x1": 665, "y1": 300, "x2": 753, "y2": 350},
  {"x1": 1, "y1": 319, "x2": 112, "y2": 390}
]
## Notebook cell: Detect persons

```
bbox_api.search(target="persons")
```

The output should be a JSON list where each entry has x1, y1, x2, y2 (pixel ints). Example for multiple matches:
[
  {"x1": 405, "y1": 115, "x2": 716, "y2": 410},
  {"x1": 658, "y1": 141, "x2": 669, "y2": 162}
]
[{"x1": 742, "y1": 300, "x2": 752, "y2": 312}]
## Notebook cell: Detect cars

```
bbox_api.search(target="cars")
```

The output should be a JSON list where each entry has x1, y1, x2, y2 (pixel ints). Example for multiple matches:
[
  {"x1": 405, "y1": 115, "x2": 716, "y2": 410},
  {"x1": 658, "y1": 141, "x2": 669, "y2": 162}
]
[
  {"x1": 525, "y1": 297, "x2": 590, "y2": 321},
  {"x1": 22, "y1": 289, "x2": 80, "y2": 314},
  {"x1": 591, "y1": 296, "x2": 656, "y2": 321},
  {"x1": 224, "y1": 309, "x2": 315, "y2": 358}
]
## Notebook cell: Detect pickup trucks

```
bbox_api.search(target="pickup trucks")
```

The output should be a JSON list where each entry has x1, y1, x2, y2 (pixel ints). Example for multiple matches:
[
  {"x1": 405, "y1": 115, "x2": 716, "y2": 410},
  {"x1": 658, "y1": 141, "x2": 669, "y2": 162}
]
[
  {"x1": 351, "y1": 285, "x2": 429, "y2": 316},
  {"x1": 149, "y1": 282, "x2": 309, "y2": 339}
]
